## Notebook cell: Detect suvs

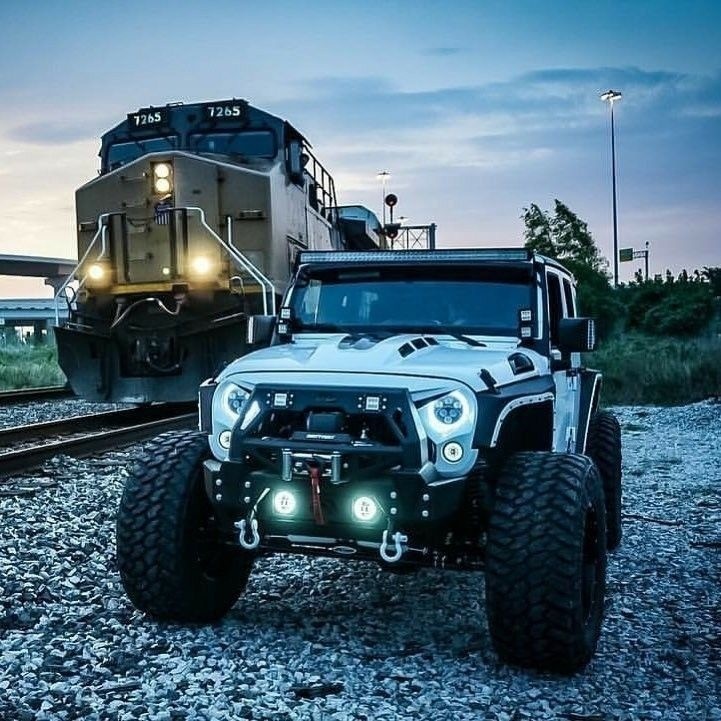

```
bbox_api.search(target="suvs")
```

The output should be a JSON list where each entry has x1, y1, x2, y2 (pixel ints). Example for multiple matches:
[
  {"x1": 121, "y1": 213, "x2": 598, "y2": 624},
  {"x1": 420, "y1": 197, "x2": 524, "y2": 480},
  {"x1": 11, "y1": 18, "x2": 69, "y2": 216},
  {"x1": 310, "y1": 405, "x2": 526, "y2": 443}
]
[{"x1": 117, "y1": 247, "x2": 623, "y2": 676}]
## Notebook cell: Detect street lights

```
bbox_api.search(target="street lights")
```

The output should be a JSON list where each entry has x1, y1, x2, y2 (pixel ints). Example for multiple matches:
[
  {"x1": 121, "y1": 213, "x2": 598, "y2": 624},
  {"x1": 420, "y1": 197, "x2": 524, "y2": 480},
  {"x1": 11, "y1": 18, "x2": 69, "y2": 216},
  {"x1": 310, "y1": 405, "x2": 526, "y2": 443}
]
[
  {"x1": 377, "y1": 171, "x2": 391, "y2": 248},
  {"x1": 599, "y1": 89, "x2": 624, "y2": 288}
]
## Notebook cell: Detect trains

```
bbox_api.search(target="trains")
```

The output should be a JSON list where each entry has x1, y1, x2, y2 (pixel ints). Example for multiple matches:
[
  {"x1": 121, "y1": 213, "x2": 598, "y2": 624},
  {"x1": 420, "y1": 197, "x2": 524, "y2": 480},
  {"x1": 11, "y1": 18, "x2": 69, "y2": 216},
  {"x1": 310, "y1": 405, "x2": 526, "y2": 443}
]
[{"x1": 54, "y1": 96, "x2": 389, "y2": 409}]
